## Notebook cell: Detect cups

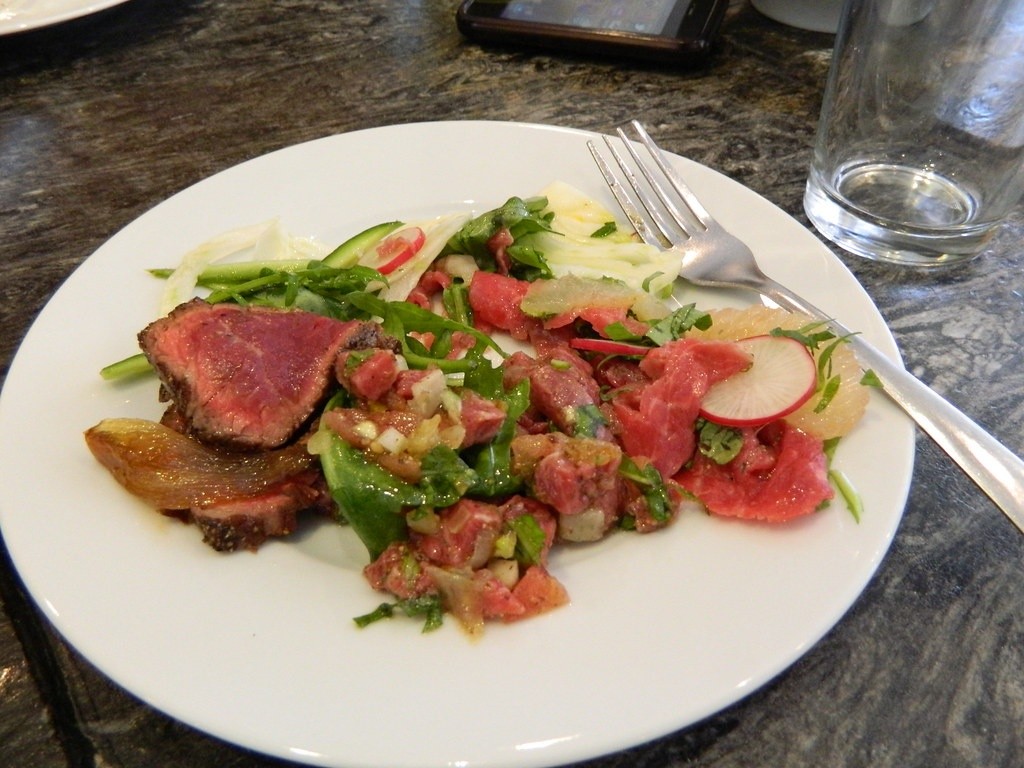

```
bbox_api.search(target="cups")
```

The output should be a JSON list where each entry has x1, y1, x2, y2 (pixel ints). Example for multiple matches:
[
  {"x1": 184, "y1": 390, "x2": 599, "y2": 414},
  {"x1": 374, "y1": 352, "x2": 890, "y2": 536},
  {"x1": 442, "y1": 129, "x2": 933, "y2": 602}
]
[{"x1": 803, "y1": 1, "x2": 1023, "y2": 269}]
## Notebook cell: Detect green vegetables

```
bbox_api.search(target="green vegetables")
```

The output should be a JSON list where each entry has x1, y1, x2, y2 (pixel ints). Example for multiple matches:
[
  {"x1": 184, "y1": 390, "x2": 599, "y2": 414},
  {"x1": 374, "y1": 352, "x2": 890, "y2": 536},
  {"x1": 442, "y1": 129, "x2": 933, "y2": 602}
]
[{"x1": 97, "y1": 193, "x2": 883, "y2": 633}]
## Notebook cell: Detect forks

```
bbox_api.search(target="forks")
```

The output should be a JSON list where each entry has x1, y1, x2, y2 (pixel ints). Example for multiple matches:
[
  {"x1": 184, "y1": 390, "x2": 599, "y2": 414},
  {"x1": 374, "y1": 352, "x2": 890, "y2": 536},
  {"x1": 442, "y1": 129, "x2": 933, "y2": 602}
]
[{"x1": 590, "y1": 116, "x2": 1023, "y2": 548}]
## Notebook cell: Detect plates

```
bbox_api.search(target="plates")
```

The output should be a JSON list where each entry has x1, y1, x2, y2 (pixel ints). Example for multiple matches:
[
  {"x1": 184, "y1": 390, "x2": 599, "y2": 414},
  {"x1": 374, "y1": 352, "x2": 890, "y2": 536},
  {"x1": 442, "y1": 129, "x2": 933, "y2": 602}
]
[{"x1": 0, "y1": 123, "x2": 915, "y2": 767}]
